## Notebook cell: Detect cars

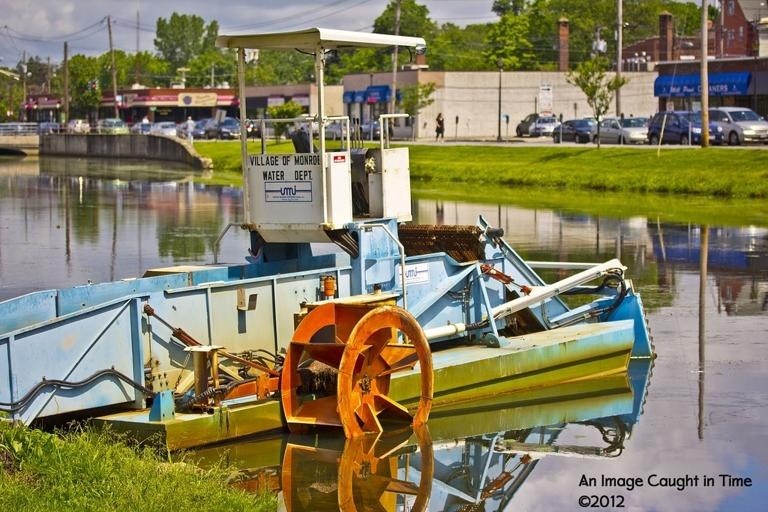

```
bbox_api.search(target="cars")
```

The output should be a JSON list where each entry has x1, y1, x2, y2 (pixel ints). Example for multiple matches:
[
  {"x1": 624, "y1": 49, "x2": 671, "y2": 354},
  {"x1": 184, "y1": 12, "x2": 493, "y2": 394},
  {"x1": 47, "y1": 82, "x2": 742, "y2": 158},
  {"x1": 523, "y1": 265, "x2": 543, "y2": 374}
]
[
  {"x1": 287, "y1": 113, "x2": 411, "y2": 139},
  {"x1": 516, "y1": 107, "x2": 767, "y2": 146},
  {"x1": 39, "y1": 116, "x2": 242, "y2": 139}
]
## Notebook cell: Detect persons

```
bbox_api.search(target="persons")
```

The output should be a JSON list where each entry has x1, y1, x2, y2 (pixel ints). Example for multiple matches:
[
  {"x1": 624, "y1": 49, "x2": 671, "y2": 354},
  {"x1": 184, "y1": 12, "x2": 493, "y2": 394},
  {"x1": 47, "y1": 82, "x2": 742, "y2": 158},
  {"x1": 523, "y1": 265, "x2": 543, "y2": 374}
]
[
  {"x1": 435, "y1": 200, "x2": 445, "y2": 225},
  {"x1": 435, "y1": 112, "x2": 446, "y2": 145},
  {"x1": 142, "y1": 114, "x2": 150, "y2": 125},
  {"x1": 185, "y1": 116, "x2": 197, "y2": 141}
]
